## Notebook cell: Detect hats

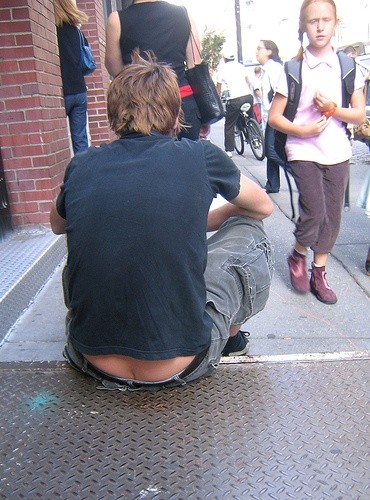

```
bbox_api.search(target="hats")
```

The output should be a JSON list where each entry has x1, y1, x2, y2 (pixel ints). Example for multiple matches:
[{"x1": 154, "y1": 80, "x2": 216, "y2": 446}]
[{"x1": 224, "y1": 49, "x2": 236, "y2": 60}]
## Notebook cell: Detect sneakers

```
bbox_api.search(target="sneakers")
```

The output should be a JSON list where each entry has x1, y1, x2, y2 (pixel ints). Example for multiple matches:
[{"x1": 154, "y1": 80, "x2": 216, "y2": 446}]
[
  {"x1": 225, "y1": 151, "x2": 232, "y2": 158},
  {"x1": 222, "y1": 330, "x2": 251, "y2": 358}
]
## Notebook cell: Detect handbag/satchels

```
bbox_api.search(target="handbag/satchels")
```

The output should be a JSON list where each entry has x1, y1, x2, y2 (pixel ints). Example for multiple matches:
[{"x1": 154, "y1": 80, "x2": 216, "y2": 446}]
[
  {"x1": 78, "y1": 29, "x2": 96, "y2": 76},
  {"x1": 185, "y1": 60, "x2": 225, "y2": 128}
]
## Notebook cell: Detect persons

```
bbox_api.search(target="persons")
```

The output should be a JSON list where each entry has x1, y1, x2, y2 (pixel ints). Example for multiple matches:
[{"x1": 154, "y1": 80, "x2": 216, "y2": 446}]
[
  {"x1": 341, "y1": 46, "x2": 359, "y2": 63},
  {"x1": 106, "y1": 0, "x2": 211, "y2": 143},
  {"x1": 214, "y1": 49, "x2": 261, "y2": 159},
  {"x1": 255, "y1": 40, "x2": 286, "y2": 195},
  {"x1": 52, "y1": 0, "x2": 89, "y2": 156},
  {"x1": 267, "y1": 0, "x2": 367, "y2": 306},
  {"x1": 49, "y1": 48, "x2": 279, "y2": 391}
]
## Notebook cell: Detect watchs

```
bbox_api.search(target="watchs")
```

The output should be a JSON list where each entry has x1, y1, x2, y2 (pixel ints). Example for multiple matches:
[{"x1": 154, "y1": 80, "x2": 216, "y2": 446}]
[{"x1": 321, "y1": 102, "x2": 337, "y2": 119}]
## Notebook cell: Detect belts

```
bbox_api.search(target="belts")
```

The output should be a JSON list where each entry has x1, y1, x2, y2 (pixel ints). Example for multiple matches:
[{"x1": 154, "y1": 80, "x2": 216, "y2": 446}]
[{"x1": 74, "y1": 336, "x2": 212, "y2": 390}]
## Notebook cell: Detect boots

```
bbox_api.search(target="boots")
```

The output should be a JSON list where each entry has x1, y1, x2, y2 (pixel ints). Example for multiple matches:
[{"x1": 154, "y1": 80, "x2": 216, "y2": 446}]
[
  {"x1": 287, "y1": 249, "x2": 310, "y2": 295},
  {"x1": 309, "y1": 262, "x2": 339, "y2": 305}
]
